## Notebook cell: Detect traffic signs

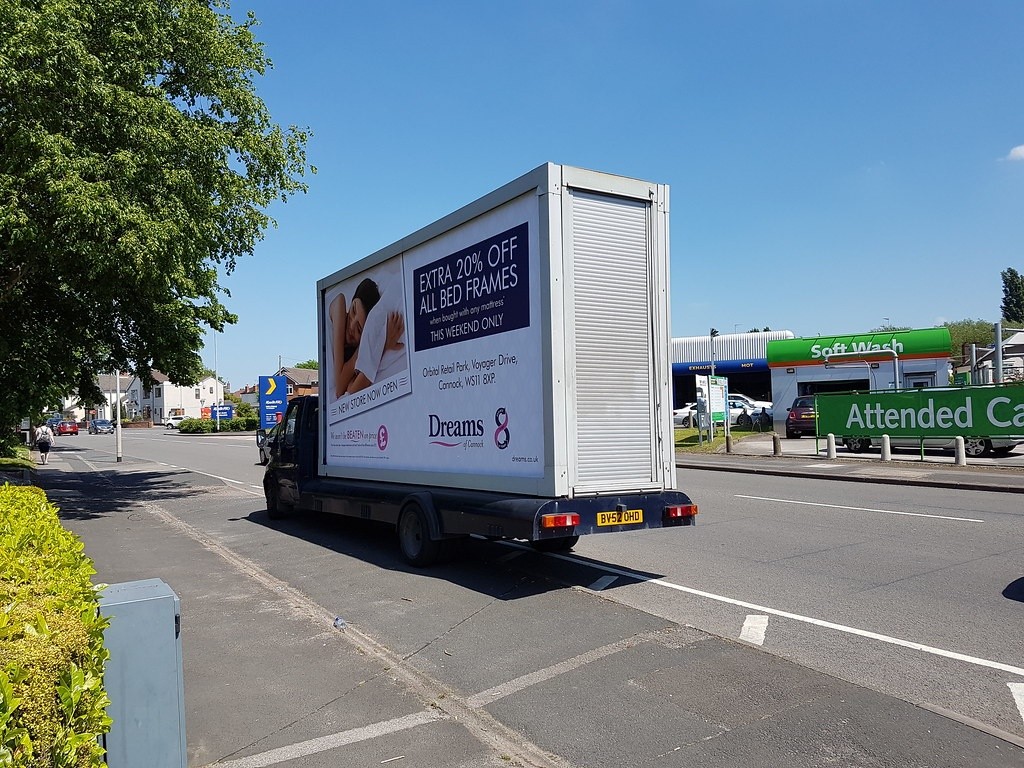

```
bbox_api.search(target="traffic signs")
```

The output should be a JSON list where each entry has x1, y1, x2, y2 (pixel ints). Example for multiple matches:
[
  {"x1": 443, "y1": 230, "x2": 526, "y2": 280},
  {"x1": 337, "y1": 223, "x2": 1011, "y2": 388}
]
[{"x1": 259, "y1": 376, "x2": 287, "y2": 430}]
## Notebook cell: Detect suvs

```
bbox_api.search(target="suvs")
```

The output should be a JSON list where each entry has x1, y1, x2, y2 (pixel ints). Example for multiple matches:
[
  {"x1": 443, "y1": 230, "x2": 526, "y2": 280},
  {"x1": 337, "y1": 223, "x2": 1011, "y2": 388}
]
[{"x1": 835, "y1": 385, "x2": 1024, "y2": 458}]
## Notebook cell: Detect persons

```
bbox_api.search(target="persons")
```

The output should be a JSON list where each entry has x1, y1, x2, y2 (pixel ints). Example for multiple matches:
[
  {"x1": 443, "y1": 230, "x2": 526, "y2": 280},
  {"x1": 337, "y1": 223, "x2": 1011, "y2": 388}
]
[
  {"x1": 321, "y1": 278, "x2": 405, "y2": 399},
  {"x1": 31, "y1": 420, "x2": 55, "y2": 465}
]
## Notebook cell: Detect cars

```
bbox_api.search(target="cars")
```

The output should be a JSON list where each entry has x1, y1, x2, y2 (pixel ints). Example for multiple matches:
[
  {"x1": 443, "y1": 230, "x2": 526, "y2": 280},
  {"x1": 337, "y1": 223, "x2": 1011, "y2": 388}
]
[
  {"x1": 88, "y1": 419, "x2": 114, "y2": 434},
  {"x1": 786, "y1": 395, "x2": 816, "y2": 439},
  {"x1": 46, "y1": 418, "x2": 79, "y2": 436},
  {"x1": 673, "y1": 393, "x2": 773, "y2": 428},
  {"x1": 260, "y1": 418, "x2": 295, "y2": 466},
  {"x1": 165, "y1": 415, "x2": 191, "y2": 429}
]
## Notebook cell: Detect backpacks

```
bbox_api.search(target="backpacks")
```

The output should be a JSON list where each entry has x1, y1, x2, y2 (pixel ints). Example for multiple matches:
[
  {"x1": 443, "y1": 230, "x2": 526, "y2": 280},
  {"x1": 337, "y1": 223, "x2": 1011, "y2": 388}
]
[{"x1": 37, "y1": 426, "x2": 50, "y2": 447}]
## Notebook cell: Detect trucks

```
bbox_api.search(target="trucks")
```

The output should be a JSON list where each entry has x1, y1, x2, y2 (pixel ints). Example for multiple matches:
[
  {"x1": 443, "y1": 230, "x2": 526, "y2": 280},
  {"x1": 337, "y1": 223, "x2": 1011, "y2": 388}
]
[{"x1": 256, "y1": 160, "x2": 698, "y2": 568}]
